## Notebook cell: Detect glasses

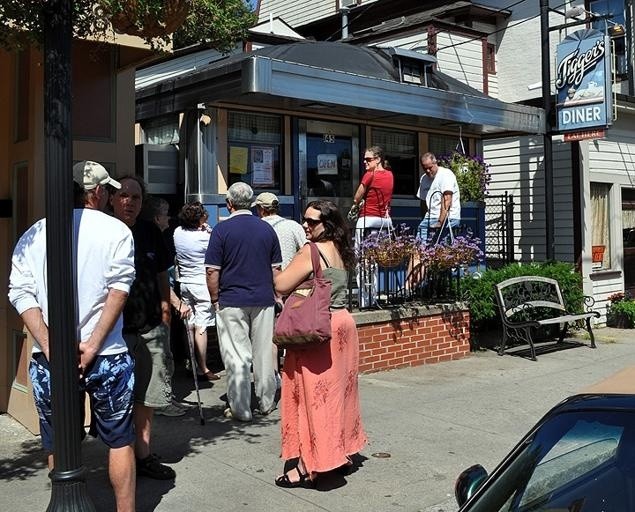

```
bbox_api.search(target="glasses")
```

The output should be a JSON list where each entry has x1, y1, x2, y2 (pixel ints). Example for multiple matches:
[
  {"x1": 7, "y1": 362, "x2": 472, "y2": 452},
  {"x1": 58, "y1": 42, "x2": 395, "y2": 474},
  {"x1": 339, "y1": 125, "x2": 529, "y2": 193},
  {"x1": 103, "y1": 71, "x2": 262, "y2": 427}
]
[
  {"x1": 365, "y1": 157, "x2": 375, "y2": 162},
  {"x1": 302, "y1": 216, "x2": 321, "y2": 227}
]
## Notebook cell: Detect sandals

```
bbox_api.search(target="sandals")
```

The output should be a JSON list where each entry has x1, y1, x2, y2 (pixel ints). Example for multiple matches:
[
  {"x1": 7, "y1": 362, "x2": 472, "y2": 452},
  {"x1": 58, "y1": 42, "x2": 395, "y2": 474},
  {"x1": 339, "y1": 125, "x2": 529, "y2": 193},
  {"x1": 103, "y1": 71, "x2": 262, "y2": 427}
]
[{"x1": 275, "y1": 465, "x2": 318, "y2": 489}]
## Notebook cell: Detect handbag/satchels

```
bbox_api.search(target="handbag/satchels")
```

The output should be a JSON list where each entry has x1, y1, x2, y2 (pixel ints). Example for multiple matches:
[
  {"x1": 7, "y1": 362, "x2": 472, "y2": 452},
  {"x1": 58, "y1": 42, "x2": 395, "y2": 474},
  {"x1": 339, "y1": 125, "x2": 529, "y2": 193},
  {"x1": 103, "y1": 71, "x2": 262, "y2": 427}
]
[
  {"x1": 273, "y1": 243, "x2": 332, "y2": 348},
  {"x1": 347, "y1": 168, "x2": 374, "y2": 224}
]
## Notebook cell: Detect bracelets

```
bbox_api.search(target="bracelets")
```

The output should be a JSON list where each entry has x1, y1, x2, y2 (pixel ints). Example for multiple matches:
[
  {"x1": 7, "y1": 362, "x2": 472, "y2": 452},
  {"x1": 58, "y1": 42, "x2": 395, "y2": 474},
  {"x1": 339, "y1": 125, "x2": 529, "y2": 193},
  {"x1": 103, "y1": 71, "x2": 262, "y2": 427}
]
[
  {"x1": 178, "y1": 300, "x2": 183, "y2": 312},
  {"x1": 437, "y1": 219, "x2": 442, "y2": 224},
  {"x1": 211, "y1": 299, "x2": 220, "y2": 304}
]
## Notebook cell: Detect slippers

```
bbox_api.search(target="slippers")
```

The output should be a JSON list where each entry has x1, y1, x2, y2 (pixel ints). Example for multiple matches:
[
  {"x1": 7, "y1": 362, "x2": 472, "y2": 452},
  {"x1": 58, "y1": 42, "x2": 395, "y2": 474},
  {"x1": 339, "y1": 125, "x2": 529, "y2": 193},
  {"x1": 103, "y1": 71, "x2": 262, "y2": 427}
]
[{"x1": 198, "y1": 370, "x2": 221, "y2": 381}]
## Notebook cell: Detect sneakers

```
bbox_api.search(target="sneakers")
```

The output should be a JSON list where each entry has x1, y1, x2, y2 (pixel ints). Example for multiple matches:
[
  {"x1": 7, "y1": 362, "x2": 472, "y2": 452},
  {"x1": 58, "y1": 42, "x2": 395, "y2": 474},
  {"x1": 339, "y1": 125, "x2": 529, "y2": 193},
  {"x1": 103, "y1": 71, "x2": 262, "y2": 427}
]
[
  {"x1": 223, "y1": 407, "x2": 233, "y2": 419},
  {"x1": 154, "y1": 399, "x2": 189, "y2": 417},
  {"x1": 136, "y1": 453, "x2": 173, "y2": 480},
  {"x1": 262, "y1": 401, "x2": 276, "y2": 415}
]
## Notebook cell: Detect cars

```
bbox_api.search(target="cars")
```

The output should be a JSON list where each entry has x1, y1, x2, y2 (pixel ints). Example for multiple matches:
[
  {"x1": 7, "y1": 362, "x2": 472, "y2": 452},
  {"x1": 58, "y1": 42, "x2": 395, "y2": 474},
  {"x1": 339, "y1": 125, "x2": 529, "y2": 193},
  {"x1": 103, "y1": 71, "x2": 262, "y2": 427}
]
[{"x1": 454, "y1": 363, "x2": 635, "y2": 512}]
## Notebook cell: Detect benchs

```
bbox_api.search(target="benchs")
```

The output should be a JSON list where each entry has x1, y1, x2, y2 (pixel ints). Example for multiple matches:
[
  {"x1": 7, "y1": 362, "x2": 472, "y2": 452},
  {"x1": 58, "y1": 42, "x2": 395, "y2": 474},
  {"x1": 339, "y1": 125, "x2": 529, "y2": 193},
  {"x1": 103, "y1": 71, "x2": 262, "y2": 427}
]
[{"x1": 490, "y1": 274, "x2": 601, "y2": 361}]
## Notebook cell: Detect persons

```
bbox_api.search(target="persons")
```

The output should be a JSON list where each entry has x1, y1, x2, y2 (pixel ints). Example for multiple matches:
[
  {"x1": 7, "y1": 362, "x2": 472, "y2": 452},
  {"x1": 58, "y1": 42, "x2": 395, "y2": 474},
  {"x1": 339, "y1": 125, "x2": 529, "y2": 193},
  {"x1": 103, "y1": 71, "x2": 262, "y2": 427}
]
[
  {"x1": 387, "y1": 151, "x2": 463, "y2": 301},
  {"x1": 204, "y1": 181, "x2": 283, "y2": 422},
  {"x1": 141, "y1": 197, "x2": 191, "y2": 419},
  {"x1": 250, "y1": 190, "x2": 314, "y2": 391},
  {"x1": 272, "y1": 199, "x2": 369, "y2": 488},
  {"x1": 172, "y1": 201, "x2": 222, "y2": 381},
  {"x1": 5, "y1": 158, "x2": 140, "y2": 512},
  {"x1": 351, "y1": 145, "x2": 396, "y2": 307},
  {"x1": 107, "y1": 174, "x2": 177, "y2": 482}
]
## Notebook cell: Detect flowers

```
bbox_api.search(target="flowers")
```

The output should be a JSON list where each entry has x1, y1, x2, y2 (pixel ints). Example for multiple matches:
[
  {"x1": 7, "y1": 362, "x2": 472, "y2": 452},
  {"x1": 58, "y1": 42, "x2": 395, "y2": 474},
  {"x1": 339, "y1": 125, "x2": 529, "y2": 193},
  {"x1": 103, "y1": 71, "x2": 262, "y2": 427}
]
[
  {"x1": 436, "y1": 149, "x2": 493, "y2": 204},
  {"x1": 351, "y1": 222, "x2": 483, "y2": 267}
]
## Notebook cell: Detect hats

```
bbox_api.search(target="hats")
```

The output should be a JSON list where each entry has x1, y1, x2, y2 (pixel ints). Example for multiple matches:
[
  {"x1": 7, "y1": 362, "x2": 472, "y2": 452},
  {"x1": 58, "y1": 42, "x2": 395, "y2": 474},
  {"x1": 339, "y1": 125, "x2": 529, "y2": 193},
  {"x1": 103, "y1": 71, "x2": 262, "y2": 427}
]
[
  {"x1": 251, "y1": 192, "x2": 280, "y2": 210},
  {"x1": 72, "y1": 159, "x2": 122, "y2": 190}
]
